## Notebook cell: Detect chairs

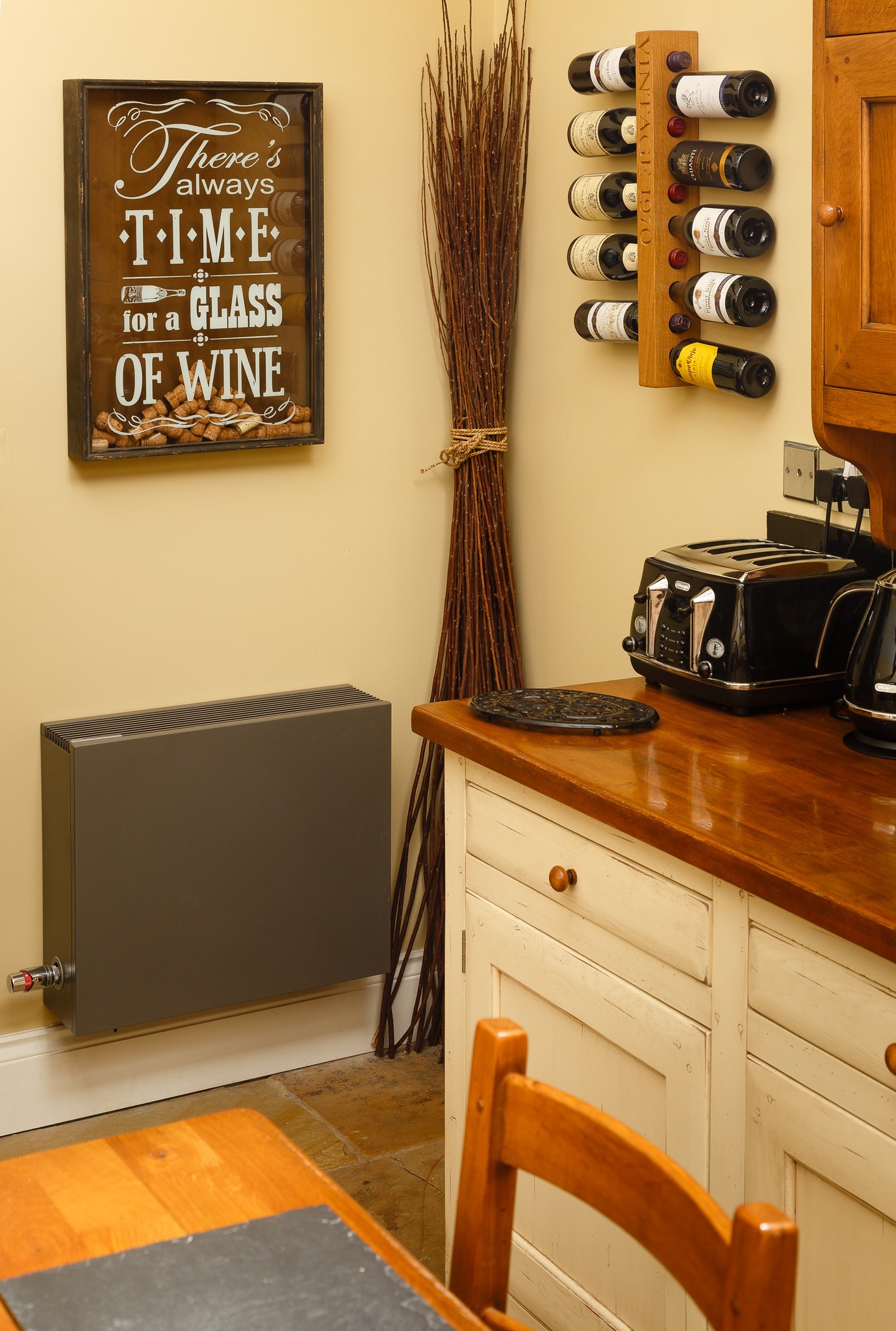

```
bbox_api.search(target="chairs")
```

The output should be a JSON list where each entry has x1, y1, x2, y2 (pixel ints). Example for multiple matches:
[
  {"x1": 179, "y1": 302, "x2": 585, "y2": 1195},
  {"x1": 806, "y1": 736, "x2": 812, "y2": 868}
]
[{"x1": 446, "y1": 1019, "x2": 808, "y2": 1330}]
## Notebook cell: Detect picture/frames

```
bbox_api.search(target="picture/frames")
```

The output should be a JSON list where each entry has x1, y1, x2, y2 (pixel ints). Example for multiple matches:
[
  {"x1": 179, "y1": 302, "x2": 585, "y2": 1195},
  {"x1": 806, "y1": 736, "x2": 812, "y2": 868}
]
[{"x1": 59, "y1": 67, "x2": 328, "y2": 460}]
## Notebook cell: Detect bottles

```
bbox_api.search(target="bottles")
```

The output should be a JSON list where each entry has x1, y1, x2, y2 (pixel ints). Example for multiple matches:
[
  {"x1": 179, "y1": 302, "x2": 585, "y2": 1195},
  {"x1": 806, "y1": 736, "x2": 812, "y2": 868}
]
[
  {"x1": 670, "y1": 271, "x2": 776, "y2": 328},
  {"x1": 567, "y1": 43, "x2": 690, "y2": 95},
  {"x1": 566, "y1": 233, "x2": 688, "y2": 282},
  {"x1": 574, "y1": 298, "x2": 691, "y2": 343},
  {"x1": 669, "y1": 204, "x2": 776, "y2": 257},
  {"x1": 667, "y1": 69, "x2": 774, "y2": 120},
  {"x1": 667, "y1": 139, "x2": 772, "y2": 191},
  {"x1": 567, "y1": 105, "x2": 687, "y2": 156},
  {"x1": 568, "y1": 171, "x2": 689, "y2": 221},
  {"x1": 668, "y1": 337, "x2": 776, "y2": 399}
]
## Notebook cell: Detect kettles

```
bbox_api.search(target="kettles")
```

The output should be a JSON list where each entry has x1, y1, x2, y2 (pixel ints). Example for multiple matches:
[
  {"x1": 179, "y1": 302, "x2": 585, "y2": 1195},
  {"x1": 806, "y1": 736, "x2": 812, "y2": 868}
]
[{"x1": 812, "y1": 568, "x2": 896, "y2": 758}]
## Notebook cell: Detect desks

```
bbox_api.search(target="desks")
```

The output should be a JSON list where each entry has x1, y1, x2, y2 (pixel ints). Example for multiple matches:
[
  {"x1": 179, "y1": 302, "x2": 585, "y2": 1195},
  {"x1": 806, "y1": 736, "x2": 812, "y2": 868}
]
[{"x1": 0, "y1": 1105, "x2": 497, "y2": 1330}]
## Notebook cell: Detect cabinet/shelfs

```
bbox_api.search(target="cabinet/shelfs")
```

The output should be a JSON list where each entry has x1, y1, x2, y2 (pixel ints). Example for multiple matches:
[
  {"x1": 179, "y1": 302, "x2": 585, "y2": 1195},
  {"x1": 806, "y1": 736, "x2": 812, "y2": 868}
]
[
  {"x1": 807, "y1": 0, "x2": 896, "y2": 435},
  {"x1": 418, "y1": 668, "x2": 896, "y2": 1330}
]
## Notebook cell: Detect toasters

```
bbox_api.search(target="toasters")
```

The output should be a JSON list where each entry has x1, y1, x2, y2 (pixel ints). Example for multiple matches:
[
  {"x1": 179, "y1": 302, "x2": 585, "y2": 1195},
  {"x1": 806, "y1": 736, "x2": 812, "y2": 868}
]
[{"x1": 623, "y1": 524, "x2": 866, "y2": 717}]
